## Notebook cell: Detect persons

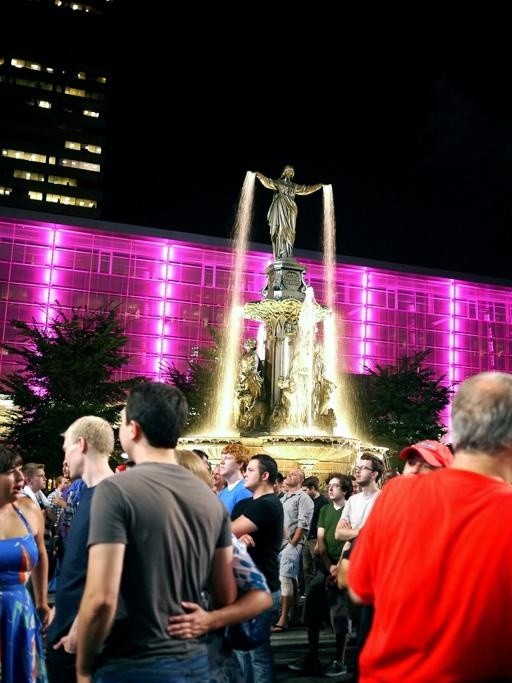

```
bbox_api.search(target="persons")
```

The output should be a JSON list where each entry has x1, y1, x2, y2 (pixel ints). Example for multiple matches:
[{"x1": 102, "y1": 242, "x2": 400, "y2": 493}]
[
  {"x1": 1, "y1": 371, "x2": 512, "y2": 682},
  {"x1": 255, "y1": 164, "x2": 321, "y2": 260},
  {"x1": 234, "y1": 337, "x2": 263, "y2": 428}
]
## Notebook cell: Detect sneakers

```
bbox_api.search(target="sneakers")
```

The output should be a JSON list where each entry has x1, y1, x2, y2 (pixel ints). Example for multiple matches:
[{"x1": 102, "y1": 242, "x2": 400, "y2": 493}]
[
  {"x1": 324, "y1": 659, "x2": 349, "y2": 678},
  {"x1": 289, "y1": 653, "x2": 322, "y2": 671}
]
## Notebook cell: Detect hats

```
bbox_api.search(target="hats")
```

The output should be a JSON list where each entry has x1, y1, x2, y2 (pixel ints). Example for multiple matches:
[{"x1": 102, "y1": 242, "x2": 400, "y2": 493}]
[{"x1": 399, "y1": 438, "x2": 455, "y2": 468}]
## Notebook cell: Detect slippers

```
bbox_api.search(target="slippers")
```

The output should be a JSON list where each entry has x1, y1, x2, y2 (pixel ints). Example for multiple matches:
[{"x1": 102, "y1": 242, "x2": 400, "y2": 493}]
[{"x1": 269, "y1": 623, "x2": 292, "y2": 632}]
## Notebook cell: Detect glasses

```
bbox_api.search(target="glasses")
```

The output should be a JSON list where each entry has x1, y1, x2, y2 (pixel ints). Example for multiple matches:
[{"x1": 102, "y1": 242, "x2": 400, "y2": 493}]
[
  {"x1": 326, "y1": 484, "x2": 343, "y2": 488},
  {"x1": 354, "y1": 466, "x2": 374, "y2": 471}
]
